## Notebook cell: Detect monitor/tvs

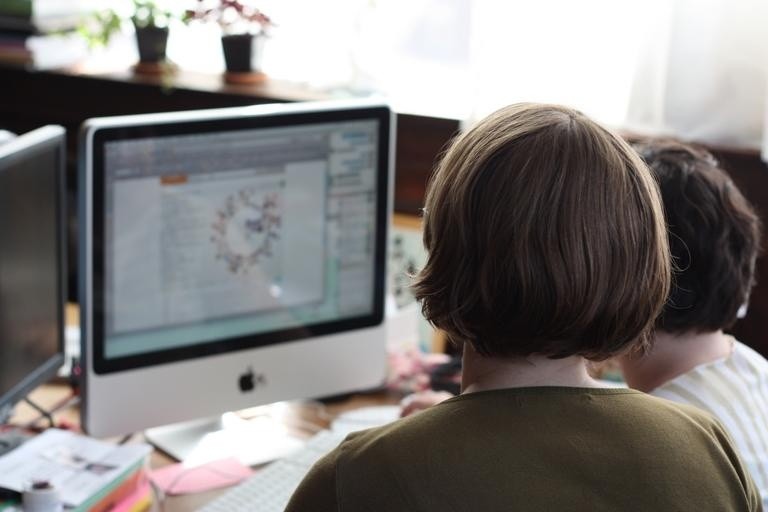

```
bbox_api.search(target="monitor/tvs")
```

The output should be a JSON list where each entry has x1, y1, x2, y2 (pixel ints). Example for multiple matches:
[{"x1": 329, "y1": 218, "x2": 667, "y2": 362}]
[
  {"x1": 78, "y1": 98, "x2": 399, "y2": 468},
  {"x1": 0, "y1": 124, "x2": 69, "y2": 455}
]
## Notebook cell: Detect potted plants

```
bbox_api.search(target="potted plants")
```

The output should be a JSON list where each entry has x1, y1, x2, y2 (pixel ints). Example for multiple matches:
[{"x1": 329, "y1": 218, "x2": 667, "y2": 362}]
[
  {"x1": 74, "y1": 5, "x2": 189, "y2": 76},
  {"x1": 193, "y1": 0, "x2": 274, "y2": 84}
]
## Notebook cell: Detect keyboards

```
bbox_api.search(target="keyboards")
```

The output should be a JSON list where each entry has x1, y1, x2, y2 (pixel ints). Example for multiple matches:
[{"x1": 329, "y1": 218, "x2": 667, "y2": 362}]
[{"x1": 197, "y1": 429, "x2": 347, "y2": 512}]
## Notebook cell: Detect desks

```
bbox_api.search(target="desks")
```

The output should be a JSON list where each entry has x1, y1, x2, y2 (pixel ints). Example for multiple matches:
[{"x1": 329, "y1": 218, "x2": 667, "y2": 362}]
[{"x1": 1, "y1": 208, "x2": 463, "y2": 510}]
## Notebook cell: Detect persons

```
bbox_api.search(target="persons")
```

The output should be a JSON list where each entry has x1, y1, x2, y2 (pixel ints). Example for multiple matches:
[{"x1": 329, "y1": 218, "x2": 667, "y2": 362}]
[
  {"x1": 283, "y1": 100, "x2": 762, "y2": 512},
  {"x1": 395, "y1": 138, "x2": 767, "y2": 510}
]
